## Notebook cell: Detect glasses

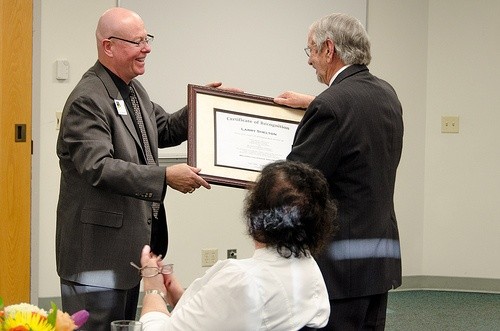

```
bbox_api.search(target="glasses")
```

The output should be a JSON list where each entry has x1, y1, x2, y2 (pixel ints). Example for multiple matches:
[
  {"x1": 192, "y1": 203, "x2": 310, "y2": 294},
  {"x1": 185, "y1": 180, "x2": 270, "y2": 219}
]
[
  {"x1": 107, "y1": 34, "x2": 155, "y2": 46},
  {"x1": 130, "y1": 254, "x2": 174, "y2": 277},
  {"x1": 305, "y1": 41, "x2": 325, "y2": 57}
]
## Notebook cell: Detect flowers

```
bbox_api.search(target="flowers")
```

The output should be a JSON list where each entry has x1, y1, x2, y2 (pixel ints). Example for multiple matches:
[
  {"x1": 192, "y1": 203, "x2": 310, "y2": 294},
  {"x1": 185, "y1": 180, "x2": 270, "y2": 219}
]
[{"x1": 0, "y1": 296, "x2": 90, "y2": 331}]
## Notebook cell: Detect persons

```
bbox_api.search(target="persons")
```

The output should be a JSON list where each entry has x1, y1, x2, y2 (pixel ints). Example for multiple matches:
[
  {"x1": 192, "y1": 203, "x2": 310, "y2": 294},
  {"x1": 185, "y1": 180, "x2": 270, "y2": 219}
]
[
  {"x1": 273, "y1": 12, "x2": 404, "y2": 331},
  {"x1": 135, "y1": 160, "x2": 338, "y2": 331},
  {"x1": 56, "y1": 7, "x2": 247, "y2": 331}
]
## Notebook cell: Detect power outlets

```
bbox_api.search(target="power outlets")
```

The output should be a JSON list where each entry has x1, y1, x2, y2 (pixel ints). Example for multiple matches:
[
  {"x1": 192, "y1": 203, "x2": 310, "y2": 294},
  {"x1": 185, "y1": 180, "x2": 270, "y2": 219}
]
[{"x1": 201, "y1": 249, "x2": 218, "y2": 267}]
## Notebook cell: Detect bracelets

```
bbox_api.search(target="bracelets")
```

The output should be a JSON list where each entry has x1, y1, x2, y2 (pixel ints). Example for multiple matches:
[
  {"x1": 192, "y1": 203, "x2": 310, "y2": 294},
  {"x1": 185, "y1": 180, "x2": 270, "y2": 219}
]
[{"x1": 144, "y1": 290, "x2": 168, "y2": 305}]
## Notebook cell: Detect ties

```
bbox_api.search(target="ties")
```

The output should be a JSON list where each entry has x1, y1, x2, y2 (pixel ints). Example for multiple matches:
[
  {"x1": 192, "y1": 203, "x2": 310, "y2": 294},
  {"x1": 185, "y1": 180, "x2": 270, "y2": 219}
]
[{"x1": 128, "y1": 87, "x2": 156, "y2": 166}]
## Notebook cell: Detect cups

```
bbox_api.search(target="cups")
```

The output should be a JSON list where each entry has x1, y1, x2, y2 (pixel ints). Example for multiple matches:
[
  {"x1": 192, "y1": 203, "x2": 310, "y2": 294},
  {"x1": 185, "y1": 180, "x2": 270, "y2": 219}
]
[{"x1": 111, "y1": 320, "x2": 143, "y2": 331}]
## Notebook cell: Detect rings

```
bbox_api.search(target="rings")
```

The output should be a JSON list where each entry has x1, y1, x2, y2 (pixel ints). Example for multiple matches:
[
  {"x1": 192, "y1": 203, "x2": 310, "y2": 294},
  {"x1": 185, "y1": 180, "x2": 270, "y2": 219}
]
[{"x1": 189, "y1": 188, "x2": 194, "y2": 193}]
